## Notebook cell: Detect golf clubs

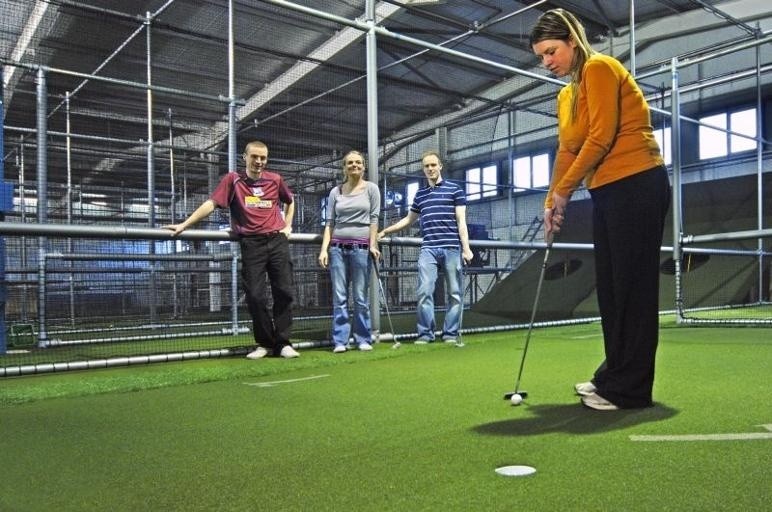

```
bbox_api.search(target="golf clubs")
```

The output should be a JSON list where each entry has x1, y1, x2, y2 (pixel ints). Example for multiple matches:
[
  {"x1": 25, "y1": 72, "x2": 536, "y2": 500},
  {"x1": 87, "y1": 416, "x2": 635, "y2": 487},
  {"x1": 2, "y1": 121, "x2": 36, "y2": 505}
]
[
  {"x1": 370, "y1": 252, "x2": 401, "y2": 349},
  {"x1": 455, "y1": 263, "x2": 467, "y2": 346},
  {"x1": 504, "y1": 208, "x2": 559, "y2": 399}
]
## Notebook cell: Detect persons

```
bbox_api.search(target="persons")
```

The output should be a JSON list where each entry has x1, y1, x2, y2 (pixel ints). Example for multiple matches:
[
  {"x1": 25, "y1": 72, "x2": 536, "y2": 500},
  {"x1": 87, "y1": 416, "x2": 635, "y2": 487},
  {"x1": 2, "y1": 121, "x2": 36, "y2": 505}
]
[
  {"x1": 527, "y1": 7, "x2": 671, "y2": 410},
  {"x1": 378, "y1": 152, "x2": 473, "y2": 346},
  {"x1": 160, "y1": 140, "x2": 299, "y2": 360},
  {"x1": 318, "y1": 151, "x2": 381, "y2": 354}
]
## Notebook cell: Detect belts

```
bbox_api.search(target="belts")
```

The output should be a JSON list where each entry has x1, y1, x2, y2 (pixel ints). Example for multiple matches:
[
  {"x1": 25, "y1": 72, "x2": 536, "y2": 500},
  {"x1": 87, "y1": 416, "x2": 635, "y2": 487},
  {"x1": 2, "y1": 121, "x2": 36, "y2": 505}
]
[{"x1": 329, "y1": 242, "x2": 368, "y2": 250}]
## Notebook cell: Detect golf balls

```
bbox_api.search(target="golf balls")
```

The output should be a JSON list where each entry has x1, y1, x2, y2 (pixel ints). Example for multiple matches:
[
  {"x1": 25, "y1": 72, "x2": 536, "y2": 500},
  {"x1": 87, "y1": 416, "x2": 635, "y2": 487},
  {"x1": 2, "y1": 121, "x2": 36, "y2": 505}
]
[{"x1": 511, "y1": 393, "x2": 522, "y2": 405}]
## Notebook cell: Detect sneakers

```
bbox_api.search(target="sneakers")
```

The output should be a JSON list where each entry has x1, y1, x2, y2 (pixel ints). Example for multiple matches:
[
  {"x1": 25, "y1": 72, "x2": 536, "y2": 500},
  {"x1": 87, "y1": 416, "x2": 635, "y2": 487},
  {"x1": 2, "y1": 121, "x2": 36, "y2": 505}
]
[
  {"x1": 414, "y1": 337, "x2": 430, "y2": 345},
  {"x1": 443, "y1": 339, "x2": 457, "y2": 344},
  {"x1": 279, "y1": 344, "x2": 300, "y2": 359},
  {"x1": 333, "y1": 343, "x2": 347, "y2": 354},
  {"x1": 245, "y1": 345, "x2": 274, "y2": 360},
  {"x1": 573, "y1": 381, "x2": 598, "y2": 397},
  {"x1": 355, "y1": 340, "x2": 373, "y2": 352},
  {"x1": 580, "y1": 393, "x2": 623, "y2": 412}
]
